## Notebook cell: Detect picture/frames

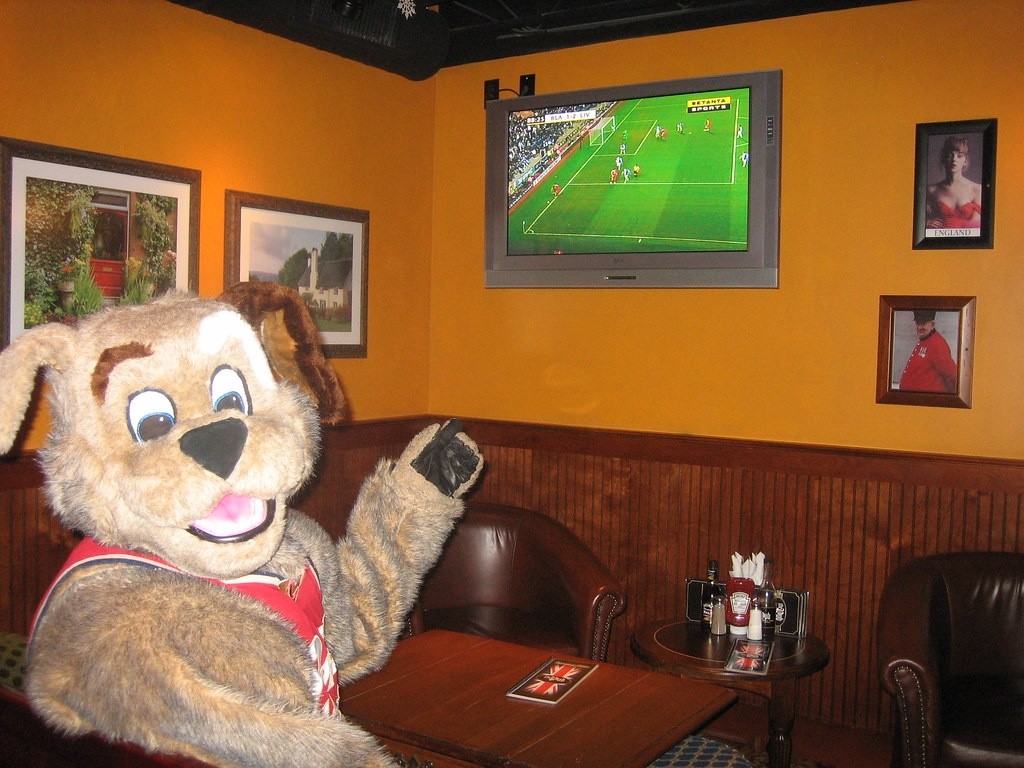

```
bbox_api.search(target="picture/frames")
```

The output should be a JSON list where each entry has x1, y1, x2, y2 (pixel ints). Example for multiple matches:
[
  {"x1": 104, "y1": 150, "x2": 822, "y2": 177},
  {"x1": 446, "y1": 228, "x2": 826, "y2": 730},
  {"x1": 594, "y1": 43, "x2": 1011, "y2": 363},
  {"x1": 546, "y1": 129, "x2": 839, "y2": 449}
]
[
  {"x1": 0, "y1": 136, "x2": 201, "y2": 354},
  {"x1": 876, "y1": 295, "x2": 977, "y2": 410},
  {"x1": 223, "y1": 188, "x2": 370, "y2": 359},
  {"x1": 912, "y1": 118, "x2": 998, "y2": 251}
]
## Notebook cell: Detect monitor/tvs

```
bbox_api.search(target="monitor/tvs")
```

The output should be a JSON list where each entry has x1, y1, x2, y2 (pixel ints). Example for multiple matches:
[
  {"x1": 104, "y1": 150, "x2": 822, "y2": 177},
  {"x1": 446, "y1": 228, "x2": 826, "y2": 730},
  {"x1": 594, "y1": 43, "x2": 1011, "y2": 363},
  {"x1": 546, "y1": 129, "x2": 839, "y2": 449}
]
[{"x1": 483, "y1": 70, "x2": 784, "y2": 289}]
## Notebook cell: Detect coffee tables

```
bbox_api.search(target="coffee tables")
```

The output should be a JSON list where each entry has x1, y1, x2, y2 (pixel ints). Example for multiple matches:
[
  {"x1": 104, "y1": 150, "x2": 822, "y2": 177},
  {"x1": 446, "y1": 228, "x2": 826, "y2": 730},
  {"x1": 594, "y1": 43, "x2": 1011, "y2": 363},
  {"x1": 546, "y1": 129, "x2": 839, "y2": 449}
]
[{"x1": 338, "y1": 629, "x2": 735, "y2": 768}]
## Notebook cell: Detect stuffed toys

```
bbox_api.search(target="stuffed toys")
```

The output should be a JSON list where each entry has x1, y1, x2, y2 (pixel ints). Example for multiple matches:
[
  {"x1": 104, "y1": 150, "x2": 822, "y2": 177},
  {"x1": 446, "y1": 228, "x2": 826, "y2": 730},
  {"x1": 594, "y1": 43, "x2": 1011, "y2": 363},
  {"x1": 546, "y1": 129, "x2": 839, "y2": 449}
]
[{"x1": 0, "y1": 274, "x2": 490, "y2": 767}]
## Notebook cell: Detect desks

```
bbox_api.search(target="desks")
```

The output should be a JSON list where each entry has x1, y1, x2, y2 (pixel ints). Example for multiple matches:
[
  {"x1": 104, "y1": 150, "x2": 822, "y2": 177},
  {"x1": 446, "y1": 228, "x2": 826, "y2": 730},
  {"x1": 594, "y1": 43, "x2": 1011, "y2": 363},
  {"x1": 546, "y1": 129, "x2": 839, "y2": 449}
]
[{"x1": 631, "y1": 617, "x2": 831, "y2": 768}]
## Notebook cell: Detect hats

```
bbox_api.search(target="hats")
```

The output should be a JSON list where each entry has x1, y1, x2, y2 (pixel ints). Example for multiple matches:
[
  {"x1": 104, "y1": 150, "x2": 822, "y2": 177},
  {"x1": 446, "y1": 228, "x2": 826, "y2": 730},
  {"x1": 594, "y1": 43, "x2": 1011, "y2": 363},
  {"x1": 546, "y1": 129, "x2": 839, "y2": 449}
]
[{"x1": 913, "y1": 311, "x2": 936, "y2": 321}]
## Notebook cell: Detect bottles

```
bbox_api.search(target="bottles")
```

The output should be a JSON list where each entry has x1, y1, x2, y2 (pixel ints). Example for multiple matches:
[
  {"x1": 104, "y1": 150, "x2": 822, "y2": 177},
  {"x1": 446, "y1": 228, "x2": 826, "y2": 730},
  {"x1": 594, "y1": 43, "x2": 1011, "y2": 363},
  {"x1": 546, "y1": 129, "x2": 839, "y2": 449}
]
[
  {"x1": 758, "y1": 559, "x2": 775, "y2": 642},
  {"x1": 747, "y1": 602, "x2": 763, "y2": 640},
  {"x1": 725, "y1": 577, "x2": 755, "y2": 635},
  {"x1": 710, "y1": 595, "x2": 726, "y2": 635},
  {"x1": 701, "y1": 560, "x2": 721, "y2": 634}
]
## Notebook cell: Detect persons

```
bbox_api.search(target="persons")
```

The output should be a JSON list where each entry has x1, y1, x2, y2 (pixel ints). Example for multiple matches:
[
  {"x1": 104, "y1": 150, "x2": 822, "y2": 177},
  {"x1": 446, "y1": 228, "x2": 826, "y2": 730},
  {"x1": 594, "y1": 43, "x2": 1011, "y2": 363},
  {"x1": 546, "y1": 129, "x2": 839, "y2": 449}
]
[
  {"x1": 927, "y1": 134, "x2": 982, "y2": 229},
  {"x1": 507, "y1": 100, "x2": 750, "y2": 197},
  {"x1": 899, "y1": 307, "x2": 960, "y2": 395}
]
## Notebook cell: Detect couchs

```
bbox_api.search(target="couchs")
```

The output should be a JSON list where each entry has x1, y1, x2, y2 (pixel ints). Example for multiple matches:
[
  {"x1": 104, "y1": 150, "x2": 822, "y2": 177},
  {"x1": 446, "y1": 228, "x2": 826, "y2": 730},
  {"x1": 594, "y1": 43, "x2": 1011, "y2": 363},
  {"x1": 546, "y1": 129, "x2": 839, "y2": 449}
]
[
  {"x1": 395, "y1": 503, "x2": 628, "y2": 662},
  {"x1": 876, "y1": 550, "x2": 1024, "y2": 768}
]
